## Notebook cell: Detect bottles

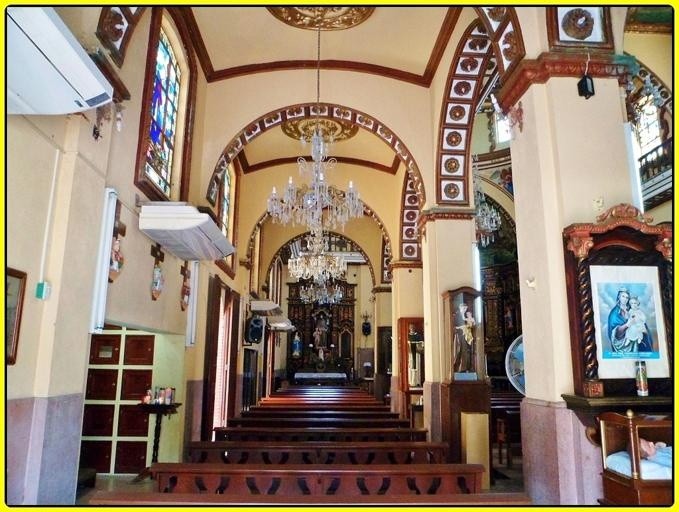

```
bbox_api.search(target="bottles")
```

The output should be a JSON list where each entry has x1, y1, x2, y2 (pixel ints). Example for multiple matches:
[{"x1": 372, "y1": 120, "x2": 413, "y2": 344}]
[
  {"x1": 635, "y1": 360, "x2": 649, "y2": 397},
  {"x1": 144, "y1": 386, "x2": 176, "y2": 404}
]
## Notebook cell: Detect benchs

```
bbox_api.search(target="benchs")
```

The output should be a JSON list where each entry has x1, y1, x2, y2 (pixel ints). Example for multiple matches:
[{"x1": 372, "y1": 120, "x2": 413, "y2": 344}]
[{"x1": 89, "y1": 384, "x2": 532, "y2": 505}]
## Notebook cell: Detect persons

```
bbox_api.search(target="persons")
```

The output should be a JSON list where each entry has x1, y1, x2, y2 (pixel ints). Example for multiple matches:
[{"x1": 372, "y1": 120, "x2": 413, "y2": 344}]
[
  {"x1": 625, "y1": 437, "x2": 672, "y2": 467},
  {"x1": 313, "y1": 328, "x2": 322, "y2": 347},
  {"x1": 505, "y1": 306, "x2": 514, "y2": 328},
  {"x1": 452, "y1": 303, "x2": 476, "y2": 374},
  {"x1": 409, "y1": 323, "x2": 424, "y2": 388},
  {"x1": 294, "y1": 331, "x2": 302, "y2": 358},
  {"x1": 607, "y1": 288, "x2": 654, "y2": 352}
]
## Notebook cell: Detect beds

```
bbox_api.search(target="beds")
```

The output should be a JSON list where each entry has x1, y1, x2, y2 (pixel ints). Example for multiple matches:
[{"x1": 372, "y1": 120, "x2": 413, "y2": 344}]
[{"x1": 597, "y1": 412, "x2": 672, "y2": 505}]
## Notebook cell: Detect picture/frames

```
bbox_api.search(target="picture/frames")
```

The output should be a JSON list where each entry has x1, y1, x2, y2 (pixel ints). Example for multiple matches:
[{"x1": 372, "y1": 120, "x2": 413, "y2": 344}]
[
  {"x1": 7, "y1": 267, "x2": 27, "y2": 365},
  {"x1": 562, "y1": 202, "x2": 673, "y2": 398}
]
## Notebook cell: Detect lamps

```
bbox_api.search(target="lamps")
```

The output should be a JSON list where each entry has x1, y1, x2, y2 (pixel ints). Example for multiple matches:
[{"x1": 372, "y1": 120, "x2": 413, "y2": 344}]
[
  {"x1": 471, "y1": 153, "x2": 501, "y2": 248},
  {"x1": 263, "y1": 7, "x2": 374, "y2": 305},
  {"x1": 577, "y1": 52, "x2": 594, "y2": 98}
]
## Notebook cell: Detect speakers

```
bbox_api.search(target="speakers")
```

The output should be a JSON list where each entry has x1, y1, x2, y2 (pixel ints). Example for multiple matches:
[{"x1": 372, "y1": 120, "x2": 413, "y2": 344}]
[
  {"x1": 245, "y1": 317, "x2": 264, "y2": 344},
  {"x1": 362, "y1": 322, "x2": 371, "y2": 335}
]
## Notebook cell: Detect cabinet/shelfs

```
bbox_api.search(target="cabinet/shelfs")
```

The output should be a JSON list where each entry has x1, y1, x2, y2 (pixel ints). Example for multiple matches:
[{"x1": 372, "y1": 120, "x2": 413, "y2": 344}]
[
  {"x1": 397, "y1": 317, "x2": 423, "y2": 429},
  {"x1": 442, "y1": 286, "x2": 492, "y2": 492}
]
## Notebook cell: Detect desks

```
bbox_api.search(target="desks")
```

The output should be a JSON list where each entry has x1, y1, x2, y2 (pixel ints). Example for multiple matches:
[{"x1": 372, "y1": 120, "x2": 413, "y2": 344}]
[{"x1": 294, "y1": 372, "x2": 346, "y2": 386}]
